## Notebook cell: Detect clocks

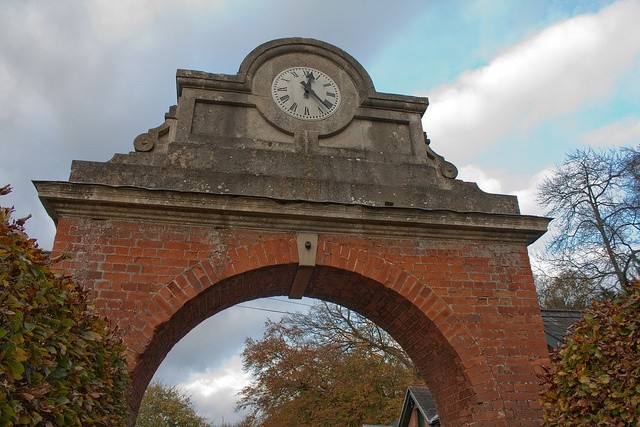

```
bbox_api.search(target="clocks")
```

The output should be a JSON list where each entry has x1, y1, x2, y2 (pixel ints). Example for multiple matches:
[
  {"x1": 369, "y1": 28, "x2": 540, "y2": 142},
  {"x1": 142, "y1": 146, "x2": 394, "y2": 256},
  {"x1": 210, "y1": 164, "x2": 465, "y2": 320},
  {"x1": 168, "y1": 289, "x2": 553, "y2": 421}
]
[{"x1": 271, "y1": 65, "x2": 342, "y2": 121}]
